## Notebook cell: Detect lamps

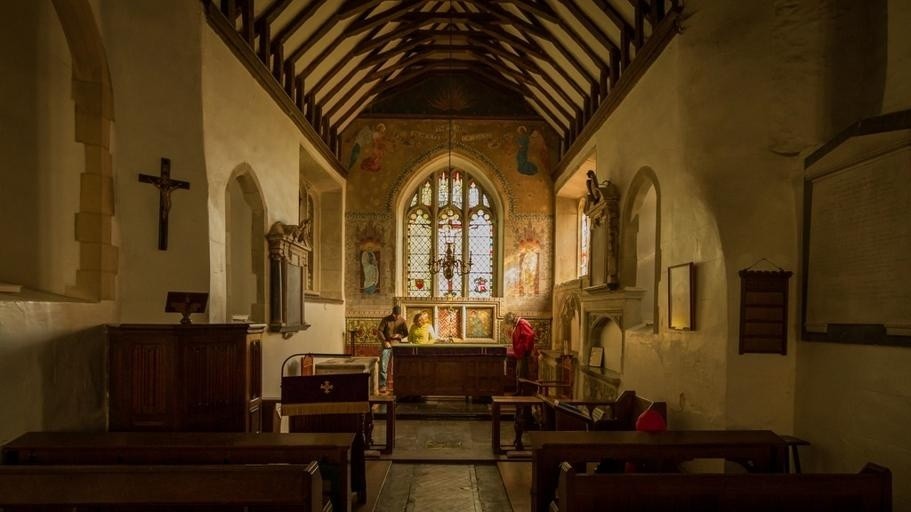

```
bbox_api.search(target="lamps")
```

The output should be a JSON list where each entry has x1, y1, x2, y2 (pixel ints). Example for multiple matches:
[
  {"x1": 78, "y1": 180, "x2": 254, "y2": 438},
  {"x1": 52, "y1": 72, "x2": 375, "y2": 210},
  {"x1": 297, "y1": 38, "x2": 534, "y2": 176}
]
[{"x1": 426, "y1": 33, "x2": 471, "y2": 281}]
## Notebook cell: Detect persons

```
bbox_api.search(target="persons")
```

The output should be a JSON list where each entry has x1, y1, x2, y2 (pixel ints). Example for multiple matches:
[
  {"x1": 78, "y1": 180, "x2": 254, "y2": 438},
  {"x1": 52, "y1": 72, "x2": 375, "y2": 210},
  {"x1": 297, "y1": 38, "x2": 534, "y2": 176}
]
[
  {"x1": 449, "y1": 318, "x2": 454, "y2": 336},
  {"x1": 148, "y1": 178, "x2": 182, "y2": 224},
  {"x1": 408, "y1": 314, "x2": 435, "y2": 344},
  {"x1": 362, "y1": 246, "x2": 379, "y2": 294},
  {"x1": 378, "y1": 306, "x2": 409, "y2": 393},
  {"x1": 504, "y1": 311, "x2": 534, "y2": 396},
  {"x1": 516, "y1": 126, "x2": 536, "y2": 175},
  {"x1": 360, "y1": 124, "x2": 391, "y2": 172}
]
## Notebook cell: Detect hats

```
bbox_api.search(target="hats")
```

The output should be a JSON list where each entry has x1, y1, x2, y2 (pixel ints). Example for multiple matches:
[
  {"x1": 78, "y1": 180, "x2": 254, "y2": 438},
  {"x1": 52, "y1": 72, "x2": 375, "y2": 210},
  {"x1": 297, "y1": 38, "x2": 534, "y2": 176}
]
[{"x1": 393, "y1": 305, "x2": 401, "y2": 314}]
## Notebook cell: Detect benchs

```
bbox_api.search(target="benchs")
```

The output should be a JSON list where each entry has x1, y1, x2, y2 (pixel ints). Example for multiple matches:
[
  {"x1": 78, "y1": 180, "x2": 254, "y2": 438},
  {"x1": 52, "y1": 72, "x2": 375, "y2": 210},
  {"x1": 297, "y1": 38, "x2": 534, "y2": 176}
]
[
  {"x1": 780, "y1": 434, "x2": 810, "y2": 473},
  {"x1": 548, "y1": 458, "x2": 893, "y2": 512}
]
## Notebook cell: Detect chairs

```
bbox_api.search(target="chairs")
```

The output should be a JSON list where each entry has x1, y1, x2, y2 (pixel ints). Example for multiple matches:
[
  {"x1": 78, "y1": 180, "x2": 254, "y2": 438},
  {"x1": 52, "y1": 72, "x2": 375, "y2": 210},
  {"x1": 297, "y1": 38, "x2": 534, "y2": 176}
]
[{"x1": 594, "y1": 390, "x2": 667, "y2": 432}]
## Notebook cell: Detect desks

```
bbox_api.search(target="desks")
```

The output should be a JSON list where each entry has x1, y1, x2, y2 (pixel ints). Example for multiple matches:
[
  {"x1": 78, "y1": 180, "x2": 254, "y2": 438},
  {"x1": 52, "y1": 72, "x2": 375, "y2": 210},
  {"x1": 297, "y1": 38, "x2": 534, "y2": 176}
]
[
  {"x1": 492, "y1": 392, "x2": 546, "y2": 449},
  {"x1": 315, "y1": 355, "x2": 381, "y2": 404},
  {"x1": 368, "y1": 395, "x2": 401, "y2": 454},
  {"x1": 2, "y1": 430, "x2": 365, "y2": 512},
  {"x1": 392, "y1": 341, "x2": 509, "y2": 405},
  {"x1": 533, "y1": 429, "x2": 786, "y2": 512}
]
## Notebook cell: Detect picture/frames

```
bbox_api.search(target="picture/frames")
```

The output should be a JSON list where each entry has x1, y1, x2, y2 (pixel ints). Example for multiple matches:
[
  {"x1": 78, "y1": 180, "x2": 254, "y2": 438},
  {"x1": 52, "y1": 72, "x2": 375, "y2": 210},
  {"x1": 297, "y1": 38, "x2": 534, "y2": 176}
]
[
  {"x1": 393, "y1": 295, "x2": 501, "y2": 344},
  {"x1": 588, "y1": 344, "x2": 605, "y2": 369},
  {"x1": 667, "y1": 261, "x2": 695, "y2": 333}
]
